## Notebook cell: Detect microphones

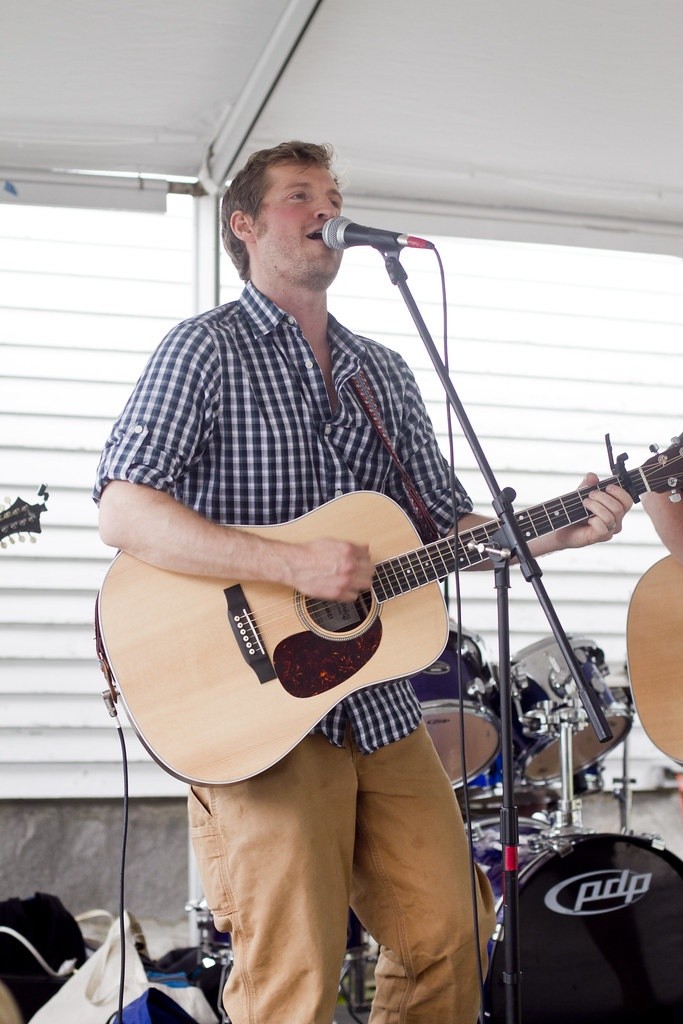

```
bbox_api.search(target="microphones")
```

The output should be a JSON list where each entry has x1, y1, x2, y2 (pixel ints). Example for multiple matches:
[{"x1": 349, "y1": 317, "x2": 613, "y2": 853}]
[{"x1": 322, "y1": 216, "x2": 435, "y2": 251}]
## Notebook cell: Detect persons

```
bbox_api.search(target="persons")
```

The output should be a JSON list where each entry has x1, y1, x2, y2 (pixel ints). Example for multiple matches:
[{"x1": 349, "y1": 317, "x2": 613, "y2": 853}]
[
  {"x1": 92, "y1": 140, "x2": 633, "y2": 1024},
  {"x1": 639, "y1": 432, "x2": 683, "y2": 564}
]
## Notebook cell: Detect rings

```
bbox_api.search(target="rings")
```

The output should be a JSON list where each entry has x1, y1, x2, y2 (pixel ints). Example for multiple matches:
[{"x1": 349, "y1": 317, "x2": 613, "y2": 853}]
[{"x1": 608, "y1": 522, "x2": 617, "y2": 530}]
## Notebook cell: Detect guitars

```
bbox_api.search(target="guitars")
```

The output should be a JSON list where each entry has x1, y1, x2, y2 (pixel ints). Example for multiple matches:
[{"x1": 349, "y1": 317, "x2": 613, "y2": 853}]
[
  {"x1": 96, "y1": 433, "x2": 683, "y2": 787},
  {"x1": 627, "y1": 553, "x2": 683, "y2": 766},
  {"x1": 0, "y1": 483, "x2": 50, "y2": 548}
]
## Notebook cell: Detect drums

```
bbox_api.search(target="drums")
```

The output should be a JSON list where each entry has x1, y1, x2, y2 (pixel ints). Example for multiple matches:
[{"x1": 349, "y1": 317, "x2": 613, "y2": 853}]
[
  {"x1": 480, "y1": 632, "x2": 635, "y2": 787},
  {"x1": 486, "y1": 826, "x2": 683, "y2": 1024},
  {"x1": 399, "y1": 618, "x2": 502, "y2": 787}
]
